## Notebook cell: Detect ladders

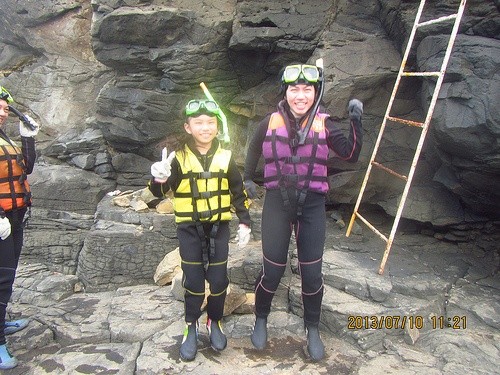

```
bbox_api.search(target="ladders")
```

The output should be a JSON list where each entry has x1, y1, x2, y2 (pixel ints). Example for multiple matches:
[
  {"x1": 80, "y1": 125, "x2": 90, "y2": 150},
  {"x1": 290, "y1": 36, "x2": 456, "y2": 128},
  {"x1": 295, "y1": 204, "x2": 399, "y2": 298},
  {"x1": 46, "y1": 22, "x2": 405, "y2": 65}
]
[{"x1": 344, "y1": 0, "x2": 467, "y2": 275}]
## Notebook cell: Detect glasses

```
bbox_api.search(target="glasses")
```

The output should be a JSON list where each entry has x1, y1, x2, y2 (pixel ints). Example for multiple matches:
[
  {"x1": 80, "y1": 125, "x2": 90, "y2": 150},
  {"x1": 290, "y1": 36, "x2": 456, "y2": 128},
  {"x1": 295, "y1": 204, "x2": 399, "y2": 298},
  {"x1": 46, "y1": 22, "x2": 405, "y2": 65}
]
[
  {"x1": 185, "y1": 99, "x2": 218, "y2": 117},
  {"x1": 0, "y1": 86, "x2": 14, "y2": 104},
  {"x1": 282, "y1": 64, "x2": 319, "y2": 85}
]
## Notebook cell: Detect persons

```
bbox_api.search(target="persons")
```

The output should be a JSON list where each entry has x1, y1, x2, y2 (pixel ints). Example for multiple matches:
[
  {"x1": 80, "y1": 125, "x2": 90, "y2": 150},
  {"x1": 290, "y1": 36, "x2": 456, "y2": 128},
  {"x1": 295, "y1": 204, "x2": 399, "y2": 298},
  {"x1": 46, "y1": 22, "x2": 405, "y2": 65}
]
[
  {"x1": 0, "y1": 86, "x2": 39, "y2": 369},
  {"x1": 243, "y1": 62, "x2": 363, "y2": 361},
  {"x1": 146, "y1": 99, "x2": 251, "y2": 362}
]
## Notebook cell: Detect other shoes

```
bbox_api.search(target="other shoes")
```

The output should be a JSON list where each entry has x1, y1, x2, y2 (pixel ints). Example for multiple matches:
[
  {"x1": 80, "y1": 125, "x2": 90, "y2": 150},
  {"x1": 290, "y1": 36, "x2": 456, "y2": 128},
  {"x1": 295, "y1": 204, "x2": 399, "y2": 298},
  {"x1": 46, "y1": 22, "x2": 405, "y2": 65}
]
[
  {"x1": 206, "y1": 317, "x2": 227, "y2": 351},
  {"x1": 252, "y1": 316, "x2": 267, "y2": 349},
  {"x1": 4, "y1": 320, "x2": 29, "y2": 335},
  {"x1": 304, "y1": 321, "x2": 325, "y2": 362},
  {"x1": 179, "y1": 321, "x2": 197, "y2": 362},
  {"x1": 0, "y1": 344, "x2": 18, "y2": 369}
]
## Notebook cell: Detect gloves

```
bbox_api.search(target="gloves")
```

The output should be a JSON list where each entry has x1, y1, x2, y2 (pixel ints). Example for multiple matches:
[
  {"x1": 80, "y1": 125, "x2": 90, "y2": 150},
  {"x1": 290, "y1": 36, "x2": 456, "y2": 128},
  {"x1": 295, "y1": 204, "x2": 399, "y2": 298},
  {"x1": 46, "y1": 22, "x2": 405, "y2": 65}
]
[
  {"x1": 20, "y1": 115, "x2": 39, "y2": 137},
  {"x1": 0, "y1": 216, "x2": 11, "y2": 240},
  {"x1": 151, "y1": 147, "x2": 176, "y2": 179},
  {"x1": 235, "y1": 223, "x2": 252, "y2": 248},
  {"x1": 244, "y1": 179, "x2": 260, "y2": 199},
  {"x1": 348, "y1": 99, "x2": 363, "y2": 119}
]
